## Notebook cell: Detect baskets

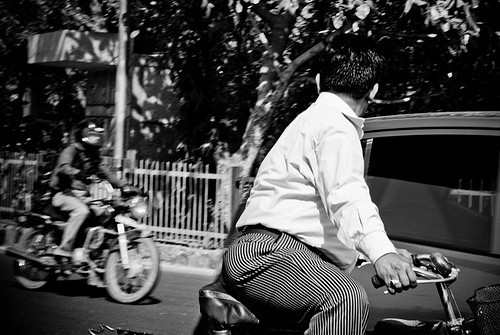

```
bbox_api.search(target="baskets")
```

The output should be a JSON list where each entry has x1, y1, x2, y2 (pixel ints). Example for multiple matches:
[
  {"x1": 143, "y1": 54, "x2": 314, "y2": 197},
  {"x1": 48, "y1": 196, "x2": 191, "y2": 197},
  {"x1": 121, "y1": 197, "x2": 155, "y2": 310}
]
[{"x1": 465, "y1": 283, "x2": 500, "y2": 335}]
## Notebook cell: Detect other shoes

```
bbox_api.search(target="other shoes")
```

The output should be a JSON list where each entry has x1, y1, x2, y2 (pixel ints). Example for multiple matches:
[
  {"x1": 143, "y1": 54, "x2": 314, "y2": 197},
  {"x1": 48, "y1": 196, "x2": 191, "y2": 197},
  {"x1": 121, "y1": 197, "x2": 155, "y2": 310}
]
[{"x1": 53, "y1": 248, "x2": 76, "y2": 258}]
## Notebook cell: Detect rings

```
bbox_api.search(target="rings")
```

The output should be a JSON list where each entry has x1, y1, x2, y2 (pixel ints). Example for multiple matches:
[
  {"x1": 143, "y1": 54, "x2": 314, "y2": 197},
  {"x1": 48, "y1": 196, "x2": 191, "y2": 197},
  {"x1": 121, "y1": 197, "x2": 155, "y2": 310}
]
[{"x1": 390, "y1": 280, "x2": 400, "y2": 287}]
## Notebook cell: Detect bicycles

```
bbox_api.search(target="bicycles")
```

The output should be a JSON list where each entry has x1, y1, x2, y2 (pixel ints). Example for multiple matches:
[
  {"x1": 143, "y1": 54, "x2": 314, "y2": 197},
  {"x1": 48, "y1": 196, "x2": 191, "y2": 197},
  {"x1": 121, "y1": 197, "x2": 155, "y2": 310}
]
[{"x1": 86, "y1": 251, "x2": 500, "y2": 335}]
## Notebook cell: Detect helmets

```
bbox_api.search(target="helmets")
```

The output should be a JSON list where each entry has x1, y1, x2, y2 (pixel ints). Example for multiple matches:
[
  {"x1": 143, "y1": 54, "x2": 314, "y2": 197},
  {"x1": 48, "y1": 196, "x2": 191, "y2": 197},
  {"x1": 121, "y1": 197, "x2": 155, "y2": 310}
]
[{"x1": 78, "y1": 117, "x2": 105, "y2": 136}]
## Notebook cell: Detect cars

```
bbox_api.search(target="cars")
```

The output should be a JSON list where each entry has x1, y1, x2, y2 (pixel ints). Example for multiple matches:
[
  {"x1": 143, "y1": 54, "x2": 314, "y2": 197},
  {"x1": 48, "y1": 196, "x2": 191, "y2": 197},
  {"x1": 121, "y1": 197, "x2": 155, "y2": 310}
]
[{"x1": 349, "y1": 110, "x2": 499, "y2": 335}]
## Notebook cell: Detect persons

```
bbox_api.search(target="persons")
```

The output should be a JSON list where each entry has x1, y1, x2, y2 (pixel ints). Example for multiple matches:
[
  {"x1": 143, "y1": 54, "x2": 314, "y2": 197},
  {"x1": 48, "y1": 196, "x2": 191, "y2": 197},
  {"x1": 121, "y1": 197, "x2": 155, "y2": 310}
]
[
  {"x1": 222, "y1": 39, "x2": 418, "y2": 335},
  {"x1": 49, "y1": 118, "x2": 142, "y2": 258}
]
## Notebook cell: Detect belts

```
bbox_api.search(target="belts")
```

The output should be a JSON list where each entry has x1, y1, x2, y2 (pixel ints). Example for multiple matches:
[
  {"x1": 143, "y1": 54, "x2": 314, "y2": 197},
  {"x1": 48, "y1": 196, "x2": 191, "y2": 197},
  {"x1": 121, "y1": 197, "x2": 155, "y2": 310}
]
[{"x1": 243, "y1": 226, "x2": 281, "y2": 238}]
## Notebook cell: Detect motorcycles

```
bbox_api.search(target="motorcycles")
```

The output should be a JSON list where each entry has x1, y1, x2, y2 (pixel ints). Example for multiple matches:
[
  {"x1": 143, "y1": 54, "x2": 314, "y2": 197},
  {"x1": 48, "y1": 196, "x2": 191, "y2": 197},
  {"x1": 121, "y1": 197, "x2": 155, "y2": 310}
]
[{"x1": 1, "y1": 173, "x2": 161, "y2": 304}]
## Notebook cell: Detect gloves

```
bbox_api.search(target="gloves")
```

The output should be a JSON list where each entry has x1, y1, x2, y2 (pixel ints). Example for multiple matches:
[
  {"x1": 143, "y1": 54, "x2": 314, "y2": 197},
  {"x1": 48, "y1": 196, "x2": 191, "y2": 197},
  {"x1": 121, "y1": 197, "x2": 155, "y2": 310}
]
[{"x1": 77, "y1": 171, "x2": 93, "y2": 185}]
